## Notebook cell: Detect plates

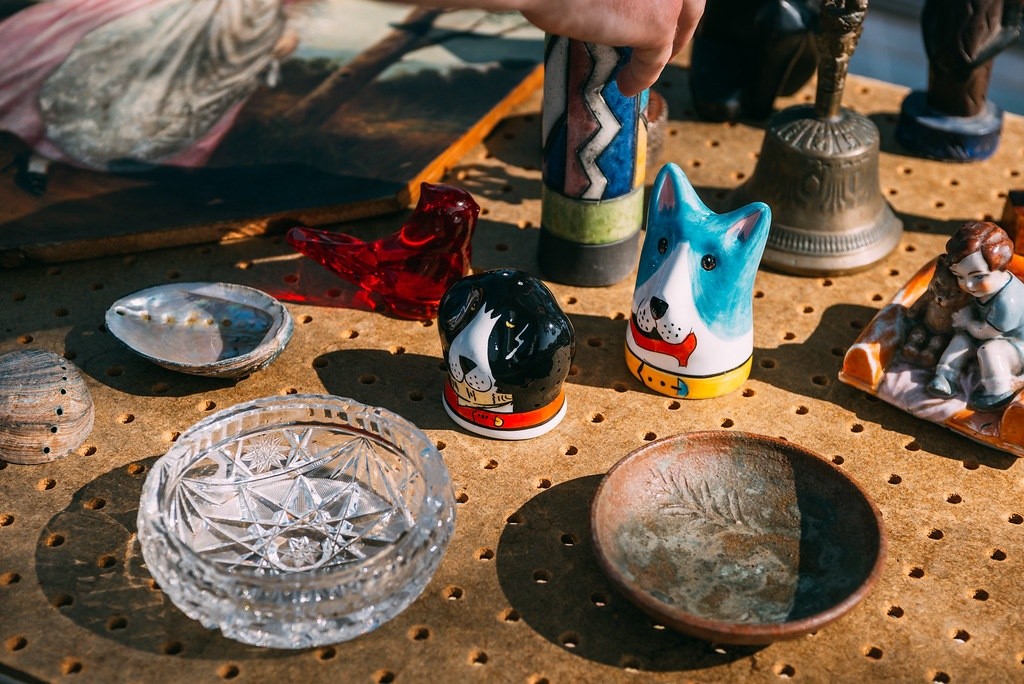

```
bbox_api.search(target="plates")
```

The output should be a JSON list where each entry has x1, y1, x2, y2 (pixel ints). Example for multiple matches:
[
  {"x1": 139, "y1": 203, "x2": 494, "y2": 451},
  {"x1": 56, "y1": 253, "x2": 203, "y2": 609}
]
[{"x1": 590, "y1": 431, "x2": 886, "y2": 644}]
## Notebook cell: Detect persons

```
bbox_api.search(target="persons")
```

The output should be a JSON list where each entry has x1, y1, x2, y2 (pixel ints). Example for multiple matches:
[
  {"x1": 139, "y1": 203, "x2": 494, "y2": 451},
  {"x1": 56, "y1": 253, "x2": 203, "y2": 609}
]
[
  {"x1": 362, "y1": 0, "x2": 705, "y2": 96},
  {"x1": 924, "y1": 221, "x2": 1024, "y2": 409}
]
